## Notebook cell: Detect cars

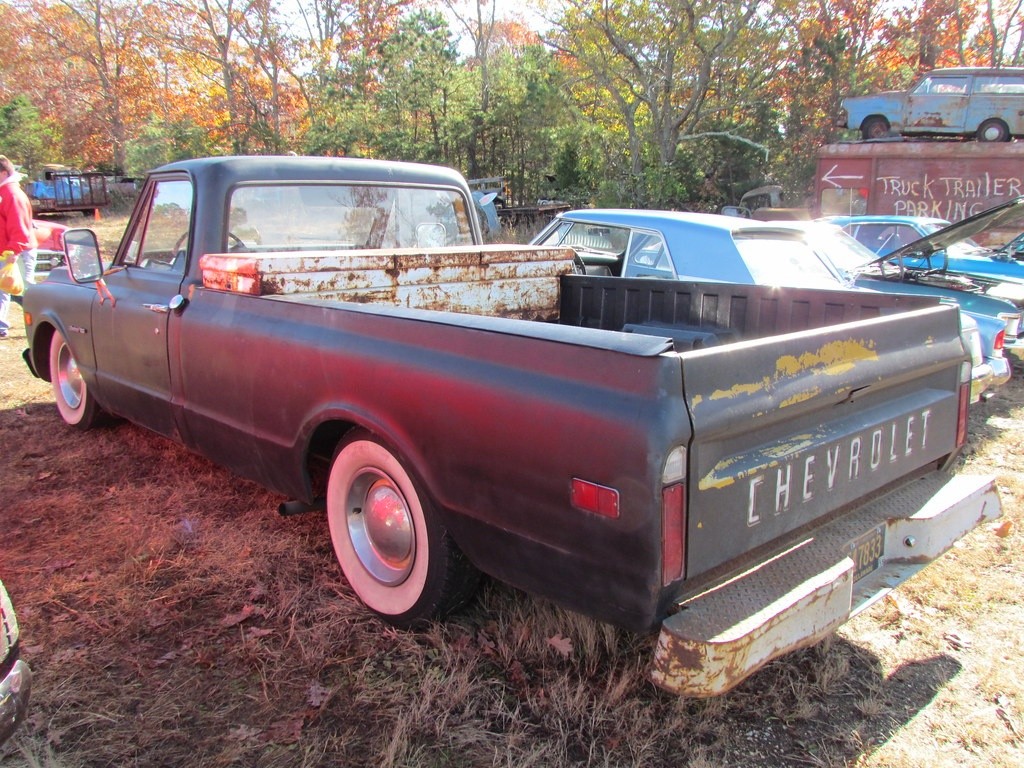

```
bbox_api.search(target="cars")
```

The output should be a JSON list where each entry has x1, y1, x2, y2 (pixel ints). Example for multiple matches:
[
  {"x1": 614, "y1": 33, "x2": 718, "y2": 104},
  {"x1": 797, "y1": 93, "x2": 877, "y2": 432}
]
[
  {"x1": 837, "y1": 66, "x2": 1024, "y2": 142},
  {"x1": 16, "y1": 165, "x2": 110, "y2": 218},
  {"x1": 372, "y1": 189, "x2": 501, "y2": 244},
  {"x1": 532, "y1": 195, "x2": 1023, "y2": 405},
  {"x1": 14, "y1": 219, "x2": 79, "y2": 277}
]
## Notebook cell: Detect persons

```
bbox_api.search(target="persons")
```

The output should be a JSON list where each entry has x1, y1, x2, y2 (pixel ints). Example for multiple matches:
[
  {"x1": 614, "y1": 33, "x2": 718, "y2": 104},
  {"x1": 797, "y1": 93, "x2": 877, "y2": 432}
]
[{"x1": 0, "y1": 155, "x2": 39, "y2": 337}]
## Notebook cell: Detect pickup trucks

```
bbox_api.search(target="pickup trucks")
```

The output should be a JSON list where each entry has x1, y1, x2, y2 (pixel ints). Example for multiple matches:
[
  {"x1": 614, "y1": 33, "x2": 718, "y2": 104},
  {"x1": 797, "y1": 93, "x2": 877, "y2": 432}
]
[{"x1": 24, "y1": 154, "x2": 1001, "y2": 699}]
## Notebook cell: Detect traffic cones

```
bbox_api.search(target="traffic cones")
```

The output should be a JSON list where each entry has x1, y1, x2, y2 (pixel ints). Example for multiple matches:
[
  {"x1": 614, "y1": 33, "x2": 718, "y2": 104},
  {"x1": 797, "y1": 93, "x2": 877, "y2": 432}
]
[{"x1": 93, "y1": 208, "x2": 103, "y2": 224}]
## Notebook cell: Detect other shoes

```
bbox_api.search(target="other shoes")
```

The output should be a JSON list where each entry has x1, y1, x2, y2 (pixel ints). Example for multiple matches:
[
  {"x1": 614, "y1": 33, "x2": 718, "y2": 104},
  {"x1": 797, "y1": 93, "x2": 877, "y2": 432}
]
[{"x1": 0, "y1": 334, "x2": 10, "y2": 342}]
